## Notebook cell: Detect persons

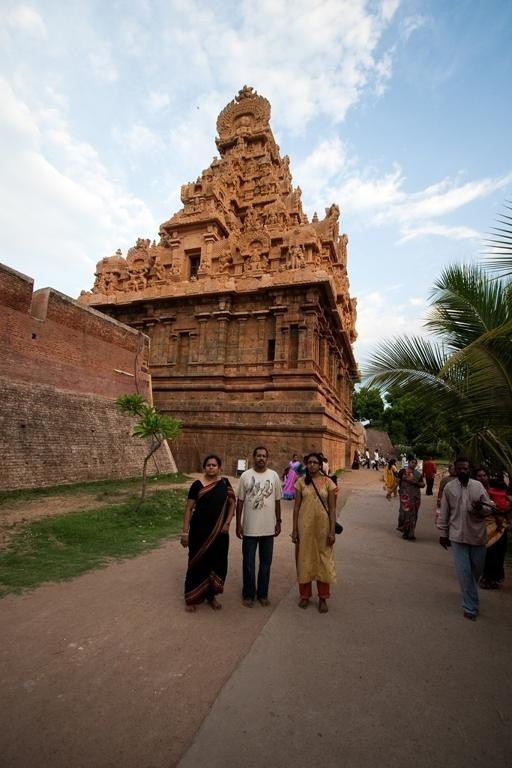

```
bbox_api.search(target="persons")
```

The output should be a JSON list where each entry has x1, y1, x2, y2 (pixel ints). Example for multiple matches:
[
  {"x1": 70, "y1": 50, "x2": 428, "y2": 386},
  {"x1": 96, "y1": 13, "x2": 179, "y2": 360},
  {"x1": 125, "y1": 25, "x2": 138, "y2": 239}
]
[
  {"x1": 291, "y1": 453, "x2": 339, "y2": 613},
  {"x1": 282, "y1": 452, "x2": 330, "y2": 500},
  {"x1": 362, "y1": 448, "x2": 511, "y2": 621},
  {"x1": 180, "y1": 455, "x2": 236, "y2": 612},
  {"x1": 236, "y1": 447, "x2": 282, "y2": 608}
]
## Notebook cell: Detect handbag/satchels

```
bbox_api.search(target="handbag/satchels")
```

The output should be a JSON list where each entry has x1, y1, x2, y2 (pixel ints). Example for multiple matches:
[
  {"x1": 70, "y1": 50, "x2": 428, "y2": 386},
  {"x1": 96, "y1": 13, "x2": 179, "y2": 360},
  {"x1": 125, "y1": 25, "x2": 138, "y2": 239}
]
[{"x1": 334, "y1": 521, "x2": 343, "y2": 534}]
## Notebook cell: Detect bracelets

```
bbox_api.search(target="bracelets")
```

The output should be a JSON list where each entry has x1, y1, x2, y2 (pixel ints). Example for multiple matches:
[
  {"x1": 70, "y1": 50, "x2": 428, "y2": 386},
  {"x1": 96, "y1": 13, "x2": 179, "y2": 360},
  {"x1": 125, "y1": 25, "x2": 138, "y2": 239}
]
[
  {"x1": 181, "y1": 533, "x2": 189, "y2": 535},
  {"x1": 277, "y1": 519, "x2": 281, "y2": 523}
]
